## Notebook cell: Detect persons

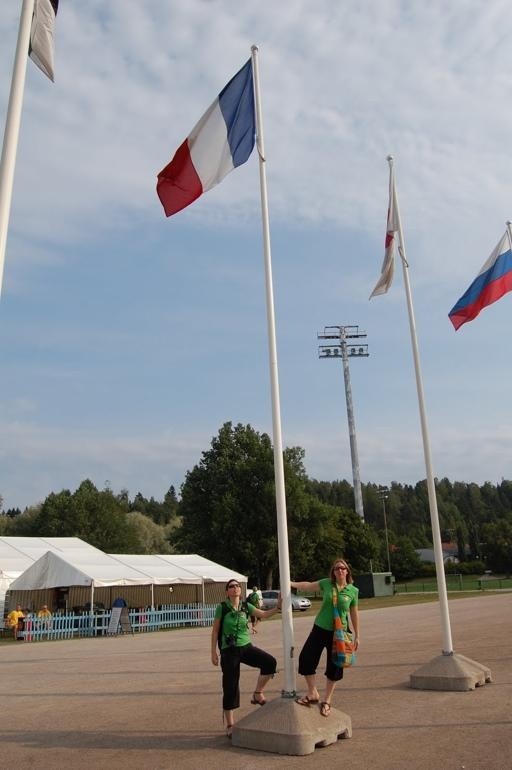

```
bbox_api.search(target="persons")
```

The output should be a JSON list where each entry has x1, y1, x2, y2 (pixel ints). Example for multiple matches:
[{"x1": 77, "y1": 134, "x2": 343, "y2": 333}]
[
  {"x1": 211, "y1": 579, "x2": 283, "y2": 739},
  {"x1": 6, "y1": 604, "x2": 24, "y2": 640},
  {"x1": 38, "y1": 605, "x2": 54, "y2": 641},
  {"x1": 291, "y1": 559, "x2": 359, "y2": 717},
  {"x1": 248, "y1": 586, "x2": 261, "y2": 634}
]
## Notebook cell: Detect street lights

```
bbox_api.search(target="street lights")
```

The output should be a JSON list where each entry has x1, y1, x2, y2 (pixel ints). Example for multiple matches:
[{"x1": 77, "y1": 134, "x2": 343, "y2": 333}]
[
  {"x1": 315, "y1": 322, "x2": 372, "y2": 570},
  {"x1": 374, "y1": 485, "x2": 394, "y2": 597}
]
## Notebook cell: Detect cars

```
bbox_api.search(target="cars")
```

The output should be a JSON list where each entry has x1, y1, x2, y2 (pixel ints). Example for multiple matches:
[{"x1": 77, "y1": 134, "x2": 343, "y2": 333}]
[{"x1": 259, "y1": 590, "x2": 314, "y2": 612}]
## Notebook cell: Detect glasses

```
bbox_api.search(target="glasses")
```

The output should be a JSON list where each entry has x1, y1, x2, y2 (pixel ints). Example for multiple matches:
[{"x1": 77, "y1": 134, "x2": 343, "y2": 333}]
[
  {"x1": 229, "y1": 584, "x2": 241, "y2": 588},
  {"x1": 333, "y1": 566, "x2": 346, "y2": 570}
]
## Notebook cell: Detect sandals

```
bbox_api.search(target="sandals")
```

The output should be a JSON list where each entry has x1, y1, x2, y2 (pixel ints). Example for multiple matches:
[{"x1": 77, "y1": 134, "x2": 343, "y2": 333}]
[
  {"x1": 251, "y1": 692, "x2": 265, "y2": 706},
  {"x1": 227, "y1": 725, "x2": 232, "y2": 739}
]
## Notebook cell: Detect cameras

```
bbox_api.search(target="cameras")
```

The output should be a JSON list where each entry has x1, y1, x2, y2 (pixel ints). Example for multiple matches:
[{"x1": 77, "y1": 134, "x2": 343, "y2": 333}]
[{"x1": 224, "y1": 633, "x2": 237, "y2": 649}]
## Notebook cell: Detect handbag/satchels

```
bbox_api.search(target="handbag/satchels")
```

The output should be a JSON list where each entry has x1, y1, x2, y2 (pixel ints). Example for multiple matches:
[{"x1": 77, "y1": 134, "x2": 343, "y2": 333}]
[{"x1": 331, "y1": 627, "x2": 355, "y2": 668}]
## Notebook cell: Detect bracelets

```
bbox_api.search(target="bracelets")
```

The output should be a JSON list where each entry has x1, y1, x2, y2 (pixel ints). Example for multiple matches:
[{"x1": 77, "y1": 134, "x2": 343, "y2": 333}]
[{"x1": 276, "y1": 606, "x2": 282, "y2": 611}]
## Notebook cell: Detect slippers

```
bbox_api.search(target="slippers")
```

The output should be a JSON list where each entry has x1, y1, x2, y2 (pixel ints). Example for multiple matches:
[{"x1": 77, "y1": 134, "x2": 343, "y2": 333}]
[
  {"x1": 321, "y1": 702, "x2": 330, "y2": 717},
  {"x1": 295, "y1": 696, "x2": 319, "y2": 705}
]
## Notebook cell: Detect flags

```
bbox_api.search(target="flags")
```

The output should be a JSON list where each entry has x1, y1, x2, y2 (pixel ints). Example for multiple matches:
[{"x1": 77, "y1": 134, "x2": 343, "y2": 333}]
[
  {"x1": 369, "y1": 164, "x2": 401, "y2": 301},
  {"x1": 156, "y1": 57, "x2": 259, "y2": 219},
  {"x1": 447, "y1": 230, "x2": 512, "y2": 332},
  {"x1": 27, "y1": 1, "x2": 62, "y2": 83}
]
